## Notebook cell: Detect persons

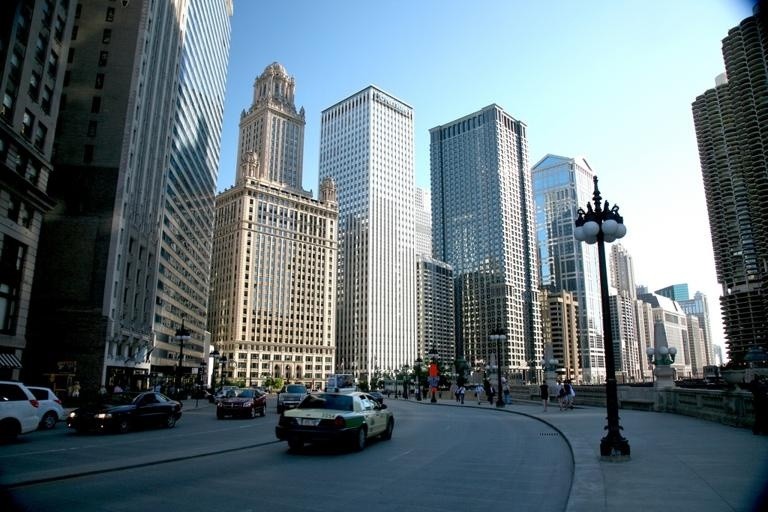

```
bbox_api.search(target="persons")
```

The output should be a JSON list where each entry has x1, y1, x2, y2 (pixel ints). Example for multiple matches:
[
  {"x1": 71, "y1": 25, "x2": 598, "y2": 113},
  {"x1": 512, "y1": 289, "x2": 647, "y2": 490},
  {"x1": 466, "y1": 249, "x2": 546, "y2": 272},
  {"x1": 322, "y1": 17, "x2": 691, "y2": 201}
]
[
  {"x1": 386, "y1": 379, "x2": 576, "y2": 412},
  {"x1": 748, "y1": 373, "x2": 766, "y2": 436},
  {"x1": 67, "y1": 378, "x2": 217, "y2": 401}
]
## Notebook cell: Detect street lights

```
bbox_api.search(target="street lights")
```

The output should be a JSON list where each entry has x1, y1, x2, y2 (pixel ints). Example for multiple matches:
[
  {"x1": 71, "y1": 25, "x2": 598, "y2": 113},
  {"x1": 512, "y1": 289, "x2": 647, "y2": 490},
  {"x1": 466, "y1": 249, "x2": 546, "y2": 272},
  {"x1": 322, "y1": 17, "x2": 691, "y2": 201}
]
[
  {"x1": 573, "y1": 174, "x2": 630, "y2": 462},
  {"x1": 173, "y1": 319, "x2": 191, "y2": 408},
  {"x1": 393, "y1": 370, "x2": 398, "y2": 398},
  {"x1": 208, "y1": 350, "x2": 220, "y2": 396},
  {"x1": 404, "y1": 365, "x2": 408, "y2": 400},
  {"x1": 414, "y1": 358, "x2": 422, "y2": 401},
  {"x1": 428, "y1": 349, "x2": 438, "y2": 402},
  {"x1": 218, "y1": 352, "x2": 227, "y2": 390},
  {"x1": 488, "y1": 323, "x2": 506, "y2": 408}
]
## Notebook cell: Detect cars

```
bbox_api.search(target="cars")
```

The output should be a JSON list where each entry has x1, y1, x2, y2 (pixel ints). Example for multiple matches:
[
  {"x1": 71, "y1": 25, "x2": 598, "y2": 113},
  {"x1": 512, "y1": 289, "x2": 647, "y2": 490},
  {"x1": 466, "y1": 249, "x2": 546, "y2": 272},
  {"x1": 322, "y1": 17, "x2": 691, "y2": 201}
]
[
  {"x1": 216, "y1": 388, "x2": 266, "y2": 420},
  {"x1": 65, "y1": 391, "x2": 182, "y2": 435},
  {"x1": 212, "y1": 386, "x2": 239, "y2": 406},
  {"x1": 0, "y1": 380, "x2": 42, "y2": 445},
  {"x1": 275, "y1": 384, "x2": 310, "y2": 414},
  {"x1": 275, "y1": 391, "x2": 394, "y2": 452},
  {"x1": 26, "y1": 386, "x2": 65, "y2": 430}
]
